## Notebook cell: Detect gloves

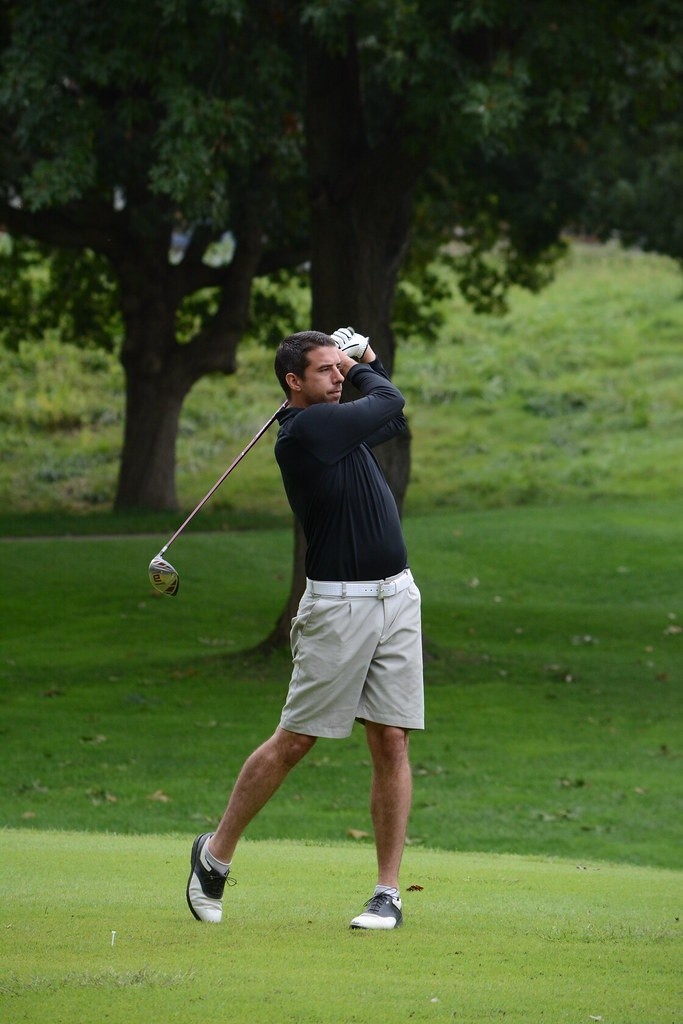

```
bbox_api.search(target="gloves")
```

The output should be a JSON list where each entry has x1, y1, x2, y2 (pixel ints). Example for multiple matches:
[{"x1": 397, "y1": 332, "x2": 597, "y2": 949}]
[{"x1": 331, "y1": 329, "x2": 369, "y2": 359}]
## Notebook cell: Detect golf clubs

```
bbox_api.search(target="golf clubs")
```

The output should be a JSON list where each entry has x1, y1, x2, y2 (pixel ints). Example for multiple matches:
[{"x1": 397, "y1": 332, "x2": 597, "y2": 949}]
[{"x1": 148, "y1": 325, "x2": 356, "y2": 598}]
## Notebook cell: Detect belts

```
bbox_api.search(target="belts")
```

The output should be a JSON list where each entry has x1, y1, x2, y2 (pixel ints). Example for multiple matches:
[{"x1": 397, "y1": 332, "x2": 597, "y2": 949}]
[{"x1": 306, "y1": 568, "x2": 410, "y2": 599}]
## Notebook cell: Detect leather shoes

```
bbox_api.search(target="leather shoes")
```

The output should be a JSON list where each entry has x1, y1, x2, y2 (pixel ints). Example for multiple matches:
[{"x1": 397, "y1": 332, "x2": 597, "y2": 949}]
[
  {"x1": 351, "y1": 889, "x2": 403, "y2": 929},
  {"x1": 186, "y1": 832, "x2": 234, "y2": 922}
]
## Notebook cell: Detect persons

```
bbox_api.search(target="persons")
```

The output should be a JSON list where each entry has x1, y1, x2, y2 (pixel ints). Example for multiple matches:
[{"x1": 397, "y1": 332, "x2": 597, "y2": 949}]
[{"x1": 185, "y1": 326, "x2": 424, "y2": 929}]
